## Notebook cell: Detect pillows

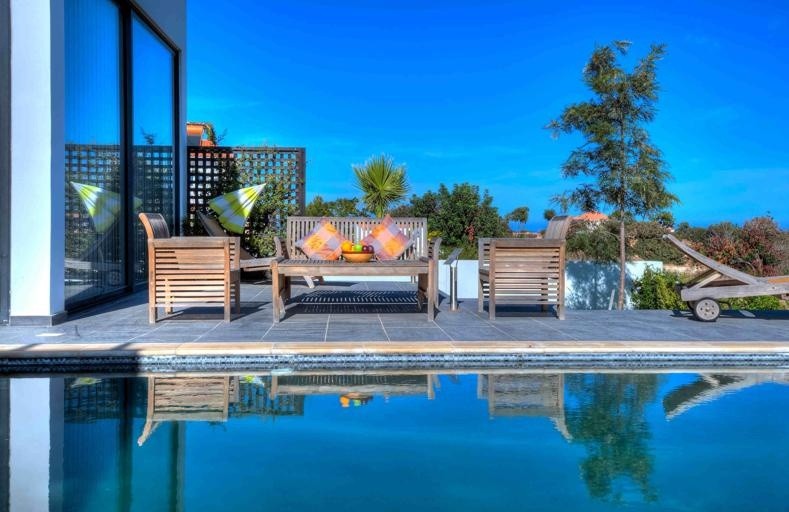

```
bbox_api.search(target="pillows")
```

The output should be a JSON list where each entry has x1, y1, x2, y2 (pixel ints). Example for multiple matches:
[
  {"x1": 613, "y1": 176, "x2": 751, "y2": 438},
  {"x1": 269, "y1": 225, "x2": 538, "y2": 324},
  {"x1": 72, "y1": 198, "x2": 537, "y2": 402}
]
[
  {"x1": 293, "y1": 216, "x2": 355, "y2": 262},
  {"x1": 356, "y1": 212, "x2": 410, "y2": 262}
]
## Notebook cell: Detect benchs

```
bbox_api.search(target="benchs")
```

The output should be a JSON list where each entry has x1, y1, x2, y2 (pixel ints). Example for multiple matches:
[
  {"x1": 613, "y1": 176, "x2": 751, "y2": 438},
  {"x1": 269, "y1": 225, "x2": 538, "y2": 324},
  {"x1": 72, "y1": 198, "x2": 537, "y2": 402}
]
[
  {"x1": 268, "y1": 372, "x2": 442, "y2": 404},
  {"x1": 268, "y1": 211, "x2": 442, "y2": 312}
]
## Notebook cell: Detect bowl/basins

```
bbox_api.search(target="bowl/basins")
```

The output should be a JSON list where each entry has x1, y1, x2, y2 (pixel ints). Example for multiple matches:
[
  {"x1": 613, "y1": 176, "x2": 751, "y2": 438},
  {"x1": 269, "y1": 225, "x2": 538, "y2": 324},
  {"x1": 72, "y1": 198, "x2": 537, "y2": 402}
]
[{"x1": 341, "y1": 251, "x2": 375, "y2": 262}]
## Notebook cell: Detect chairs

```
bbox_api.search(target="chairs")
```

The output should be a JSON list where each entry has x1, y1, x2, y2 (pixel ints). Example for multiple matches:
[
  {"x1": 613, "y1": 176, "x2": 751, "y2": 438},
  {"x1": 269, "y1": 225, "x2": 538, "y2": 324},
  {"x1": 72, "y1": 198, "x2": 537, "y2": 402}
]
[
  {"x1": 137, "y1": 372, "x2": 240, "y2": 449},
  {"x1": 135, "y1": 209, "x2": 247, "y2": 326},
  {"x1": 467, "y1": 211, "x2": 576, "y2": 322},
  {"x1": 474, "y1": 372, "x2": 577, "y2": 446},
  {"x1": 658, "y1": 232, "x2": 788, "y2": 321},
  {"x1": 662, "y1": 372, "x2": 789, "y2": 422},
  {"x1": 195, "y1": 207, "x2": 325, "y2": 291}
]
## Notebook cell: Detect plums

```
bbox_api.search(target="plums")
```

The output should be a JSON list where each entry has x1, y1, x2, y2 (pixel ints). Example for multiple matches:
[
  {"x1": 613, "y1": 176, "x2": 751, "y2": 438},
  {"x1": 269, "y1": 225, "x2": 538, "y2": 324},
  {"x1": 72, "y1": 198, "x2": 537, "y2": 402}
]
[
  {"x1": 368, "y1": 245, "x2": 374, "y2": 253},
  {"x1": 362, "y1": 245, "x2": 368, "y2": 253}
]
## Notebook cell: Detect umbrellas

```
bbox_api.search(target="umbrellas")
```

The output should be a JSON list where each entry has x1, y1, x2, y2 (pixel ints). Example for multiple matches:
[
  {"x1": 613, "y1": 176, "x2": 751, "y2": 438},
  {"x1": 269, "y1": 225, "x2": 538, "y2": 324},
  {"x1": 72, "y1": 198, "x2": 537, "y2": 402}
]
[
  {"x1": 70, "y1": 378, "x2": 102, "y2": 388},
  {"x1": 70, "y1": 180, "x2": 143, "y2": 234},
  {"x1": 242, "y1": 374, "x2": 266, "y2": 389},
  {"x1": 206, "y1": 180, "x2": 269, "y2": 235}
]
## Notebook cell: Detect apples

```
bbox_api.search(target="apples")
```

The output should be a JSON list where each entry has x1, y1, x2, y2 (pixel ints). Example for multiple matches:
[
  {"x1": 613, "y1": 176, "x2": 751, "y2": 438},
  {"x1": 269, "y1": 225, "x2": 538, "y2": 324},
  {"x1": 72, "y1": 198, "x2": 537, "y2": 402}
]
[
  {"x1": 354, "y1": 244, "x2": 362, "y2": 252},
  {"x1": 353, "y1": 400, "x2": 361, "y2": 407}
]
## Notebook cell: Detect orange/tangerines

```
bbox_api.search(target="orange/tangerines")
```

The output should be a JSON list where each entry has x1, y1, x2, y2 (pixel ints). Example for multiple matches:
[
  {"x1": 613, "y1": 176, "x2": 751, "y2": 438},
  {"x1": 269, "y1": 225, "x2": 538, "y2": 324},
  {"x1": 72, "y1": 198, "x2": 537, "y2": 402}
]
[{"x1": 339, "y1": 396, "x2": 350, "y2": 408}]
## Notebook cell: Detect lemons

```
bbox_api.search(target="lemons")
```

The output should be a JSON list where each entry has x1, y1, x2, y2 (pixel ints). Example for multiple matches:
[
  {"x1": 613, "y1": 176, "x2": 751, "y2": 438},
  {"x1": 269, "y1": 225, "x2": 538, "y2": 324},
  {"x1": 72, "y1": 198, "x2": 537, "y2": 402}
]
[{"x1": 340, "y1": 240, "x2": 352, "y2": 252}]
